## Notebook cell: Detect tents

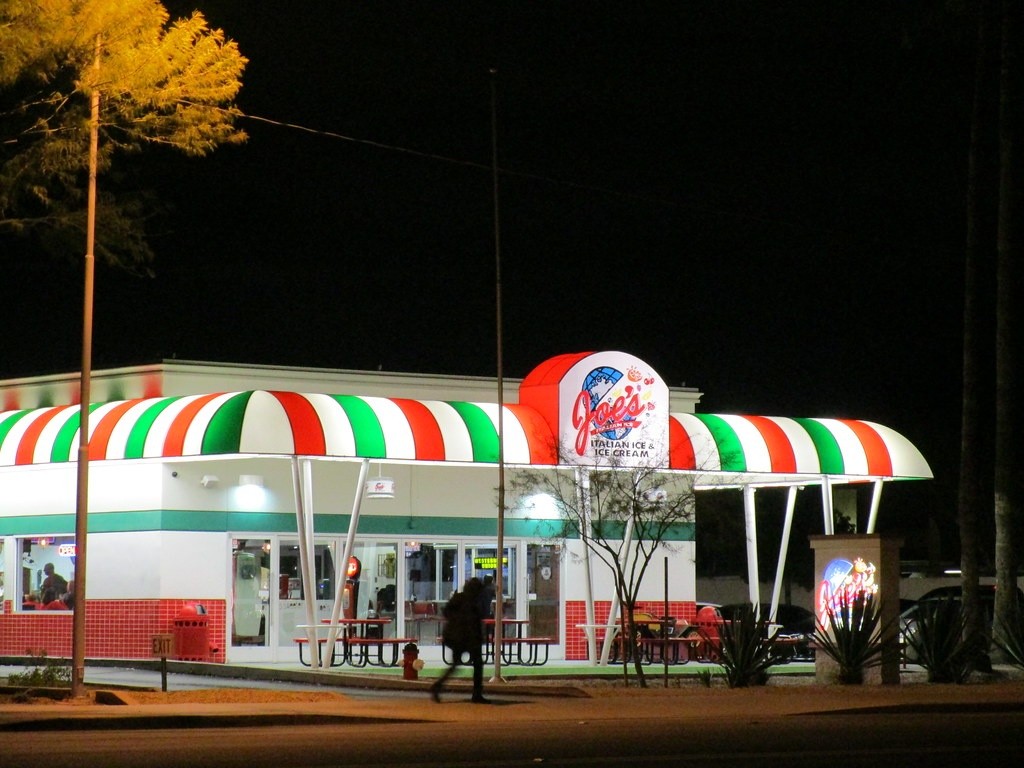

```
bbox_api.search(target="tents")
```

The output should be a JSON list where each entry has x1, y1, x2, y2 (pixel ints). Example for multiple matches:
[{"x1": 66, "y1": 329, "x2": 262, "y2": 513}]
[{"x1": 3, "y1": 345, "x2": 935, "y2": 674}]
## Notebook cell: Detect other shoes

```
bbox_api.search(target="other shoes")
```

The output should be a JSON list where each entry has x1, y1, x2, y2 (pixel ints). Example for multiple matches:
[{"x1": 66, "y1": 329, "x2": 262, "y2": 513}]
[
  {"x1": 471, "y1": 687, "x2": 490, "y2": 707},
  {"x1": 429, "y1": 680, "x2": 443, "y2": 701}
]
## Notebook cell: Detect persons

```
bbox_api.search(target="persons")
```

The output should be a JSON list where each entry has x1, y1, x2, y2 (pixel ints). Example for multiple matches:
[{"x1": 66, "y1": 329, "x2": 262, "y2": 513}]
[
  {"x1": 429, "y1": 578, "x2": 486, "y2": 703},
  {"x1": 62, "y1": 584, "x2": 75, "y2": 608},
  {"x1": 40, "y1": 586, "x2": 57, "y2": 608},
  {"x1": 40, "y1": 562, "x2": 67, "y2": 594},
  {"x1": 481, "y1": 574, "x2": 495, "y2": 609}
]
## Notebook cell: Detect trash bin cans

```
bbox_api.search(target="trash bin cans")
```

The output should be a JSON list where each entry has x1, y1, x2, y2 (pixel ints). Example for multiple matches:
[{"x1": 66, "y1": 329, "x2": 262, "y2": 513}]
[
  {"x1": 696, "y1": 606, "x2": 723, "y2": 661},
  {"x1": 173, "y1": 602, "x2": 207, "y2": 662}
]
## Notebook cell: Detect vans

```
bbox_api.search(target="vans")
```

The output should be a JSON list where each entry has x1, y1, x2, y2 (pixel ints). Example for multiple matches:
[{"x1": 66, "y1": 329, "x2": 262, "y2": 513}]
[{"x1": 900, "y1": 584, "x2": 1023, "y2": 668}]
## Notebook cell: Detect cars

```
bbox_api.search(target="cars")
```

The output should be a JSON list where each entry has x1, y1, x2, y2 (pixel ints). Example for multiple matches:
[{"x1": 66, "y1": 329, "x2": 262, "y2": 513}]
[
  {"x1": 717, "y1": 603, "x2": 826, "y2": 660},
  {"x1": 694, "y1": 600, "x2": 723, "y2": 619}
]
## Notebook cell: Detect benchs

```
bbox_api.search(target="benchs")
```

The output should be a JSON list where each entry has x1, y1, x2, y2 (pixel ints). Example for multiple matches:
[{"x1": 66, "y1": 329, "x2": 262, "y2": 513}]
[
  {"x1": 583, "y1": 618, "x2": 800, "y2": 665},
  {"x1": 436, "y1": 619, "x2": 551, "y2": 665},
  {"x1": 294, "y1": 619, "x2": 418, "y2": 666}
]
